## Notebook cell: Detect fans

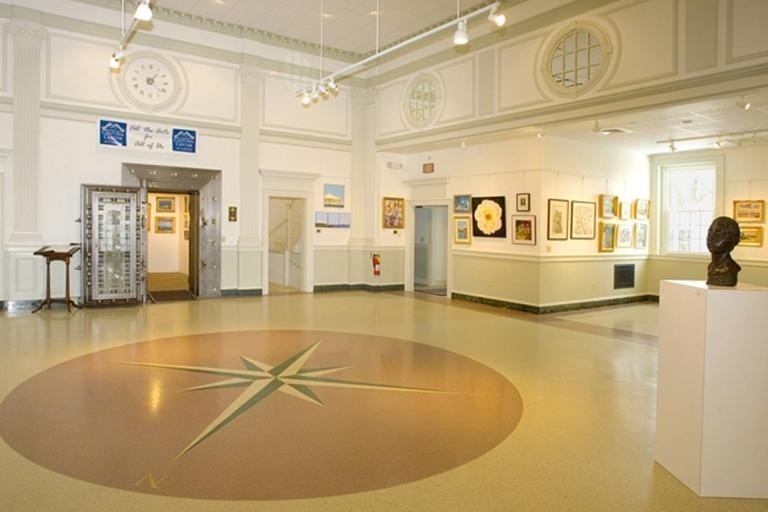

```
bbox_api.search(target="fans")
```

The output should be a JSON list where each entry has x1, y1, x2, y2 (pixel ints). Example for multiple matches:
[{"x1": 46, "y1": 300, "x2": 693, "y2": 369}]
[{"x1": 577, "y1": 118, "x2": 631, "y2": 139}]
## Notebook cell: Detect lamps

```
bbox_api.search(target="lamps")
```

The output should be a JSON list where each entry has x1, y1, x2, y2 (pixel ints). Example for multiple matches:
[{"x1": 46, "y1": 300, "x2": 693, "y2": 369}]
[
  {"x1": 292, "y1": 0, "x2": 505, "y2": 107},
  {"x1": 656, "y1": 129, "x2": 768, "y2": 150},
  {"x1": 460, "y1": 136, "x2": 471, "y2": 150},
  {"x1": 106, "y1": 0, "x2": 152, "y2": 72},
  {"x1": 736, "y1": 94, "x2": 750, "y2": 111},
  {"x1": 535, "y1": 129, "x2": 545, "y2": 146}
]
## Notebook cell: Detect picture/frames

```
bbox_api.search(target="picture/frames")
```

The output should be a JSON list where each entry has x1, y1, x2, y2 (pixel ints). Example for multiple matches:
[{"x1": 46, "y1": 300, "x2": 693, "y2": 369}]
[
  {"x1": 452, "y1": 192, "x2": 536, "y2": 246},
  {"x1": 571, "y1": 200, "x2": 596, "y2": 240},
  {"x1": 599, "y1": 193, "x2": 651, "y2": 252},
  {"x1": 736, "y1": 226, "x2": 764, "y2": 249},
  {"x1": 548, "y1": 199, "x2": 569, "y2": 240},
  {"x1": 732, "y1": 200, "x2": 764, "y2": 223},
  {"x1": 147, "y1": 196, "x2": 190, "y2": 241},
  {"x1": 382, "y1": 196, "x2": 405, "y2": 229}
]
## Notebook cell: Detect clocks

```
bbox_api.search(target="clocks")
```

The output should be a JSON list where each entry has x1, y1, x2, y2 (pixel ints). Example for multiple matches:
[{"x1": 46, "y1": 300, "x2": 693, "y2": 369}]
[{"x1": 110, "y1": 49, "x2": 189, "y2": 117}]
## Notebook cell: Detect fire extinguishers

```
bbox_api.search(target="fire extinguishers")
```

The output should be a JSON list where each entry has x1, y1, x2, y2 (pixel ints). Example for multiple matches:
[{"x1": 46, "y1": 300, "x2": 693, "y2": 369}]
[{"x1": 372, "y1": 254, "x2": 380, "y2": 275}]
